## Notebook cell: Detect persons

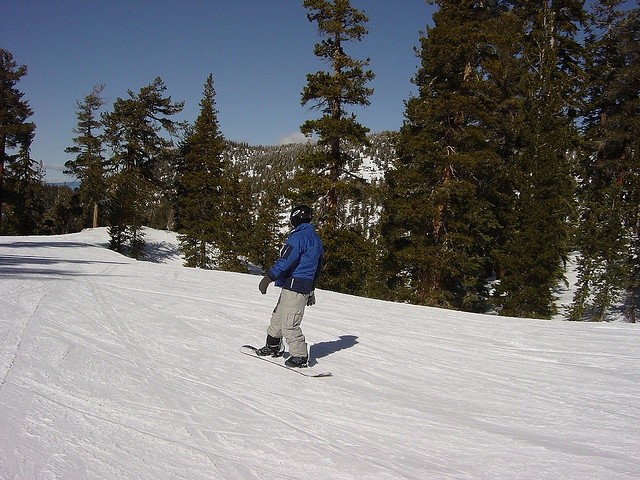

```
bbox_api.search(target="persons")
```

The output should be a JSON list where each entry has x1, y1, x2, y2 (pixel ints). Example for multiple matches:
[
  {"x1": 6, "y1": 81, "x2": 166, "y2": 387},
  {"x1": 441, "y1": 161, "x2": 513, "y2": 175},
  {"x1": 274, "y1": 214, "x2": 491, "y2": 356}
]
[{"x1": 255, "y1": 205, "x2": 325, "y2": 366}]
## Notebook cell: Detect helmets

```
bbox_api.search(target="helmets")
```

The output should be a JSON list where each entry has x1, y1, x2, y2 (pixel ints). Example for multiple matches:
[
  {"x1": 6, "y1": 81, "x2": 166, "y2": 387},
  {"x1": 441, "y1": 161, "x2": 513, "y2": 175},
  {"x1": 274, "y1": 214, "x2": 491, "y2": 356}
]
[{"x1": 290, "y1": 204, "x2": 313, "y2": 227}]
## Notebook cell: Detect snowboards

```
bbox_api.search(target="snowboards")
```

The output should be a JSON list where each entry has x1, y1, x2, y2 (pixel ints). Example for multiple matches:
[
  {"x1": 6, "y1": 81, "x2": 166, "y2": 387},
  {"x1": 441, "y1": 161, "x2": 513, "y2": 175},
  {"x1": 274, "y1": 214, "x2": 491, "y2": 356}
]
[{"x1": 240, "y1": 345, "x2": 332, "y2": 377}]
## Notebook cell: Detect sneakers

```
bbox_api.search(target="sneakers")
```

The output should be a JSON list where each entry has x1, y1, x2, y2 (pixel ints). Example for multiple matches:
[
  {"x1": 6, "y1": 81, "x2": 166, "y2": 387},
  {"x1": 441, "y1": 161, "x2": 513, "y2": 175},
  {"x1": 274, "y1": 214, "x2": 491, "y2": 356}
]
[
  {"x1": 285, "y1": 357, "x2": 307, "y2": 367},
  {"x1": 256, "y1": 345, "x2": 281, "y2": 356}
]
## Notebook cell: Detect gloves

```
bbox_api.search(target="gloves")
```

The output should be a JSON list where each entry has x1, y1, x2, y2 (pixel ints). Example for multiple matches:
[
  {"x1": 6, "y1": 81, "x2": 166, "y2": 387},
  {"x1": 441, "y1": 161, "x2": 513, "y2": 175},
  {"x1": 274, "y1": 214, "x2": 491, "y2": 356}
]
[
  {"x1": 307, "y1": 291, "x2": 316, "y2": 306},
  {"x1": 259, "y1": 273, "x2": 272, "y2": 294}
]
